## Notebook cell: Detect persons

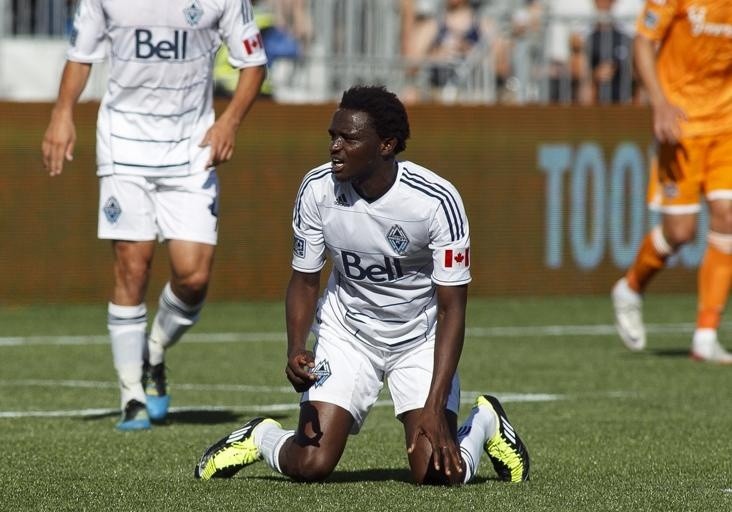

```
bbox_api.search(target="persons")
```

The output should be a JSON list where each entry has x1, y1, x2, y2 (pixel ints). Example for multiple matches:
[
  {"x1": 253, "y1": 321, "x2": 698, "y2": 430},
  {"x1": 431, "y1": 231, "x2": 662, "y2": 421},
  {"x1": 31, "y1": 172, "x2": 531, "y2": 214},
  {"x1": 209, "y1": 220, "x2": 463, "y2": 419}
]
[
  {"x1": 604, "y1": 0, "x2": 732, "y2": 365},
  {"x1": 40, "y1": 1, "x2": 269, "y2": 431},
  {"x1": 195, "y1": 85, "x2": 529, "y2": 483},
  {"x1": 204, "y1": 2, "x2": 647, "y2": 105}
]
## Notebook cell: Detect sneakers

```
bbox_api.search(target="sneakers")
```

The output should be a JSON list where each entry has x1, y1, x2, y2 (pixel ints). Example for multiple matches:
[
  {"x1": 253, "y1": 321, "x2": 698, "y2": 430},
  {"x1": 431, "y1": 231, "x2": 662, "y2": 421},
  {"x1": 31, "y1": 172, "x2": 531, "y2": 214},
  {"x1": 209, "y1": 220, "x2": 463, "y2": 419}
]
[
  {"x1": 690, "y1": 333, "x2": 732, "y2": 366},
  {"x1": 612, "y1": 277, "x2": 646, "y2": 352},
  {"x1": 195, "y1": 417, "x2": 282, "y2": 480},
  {"x1": 471, "y1": 395, "x2": 529, "y2": 483},
  {"x1": 116, "y1": 347, "x2": 168, "y2": 431}
]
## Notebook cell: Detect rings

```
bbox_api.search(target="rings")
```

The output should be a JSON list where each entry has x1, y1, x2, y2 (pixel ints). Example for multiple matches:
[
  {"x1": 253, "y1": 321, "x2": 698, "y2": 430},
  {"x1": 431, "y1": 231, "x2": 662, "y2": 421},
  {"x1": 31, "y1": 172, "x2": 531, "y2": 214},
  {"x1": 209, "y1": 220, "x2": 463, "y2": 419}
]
[{"x1": 442, "y1": 446, "x2": 448, "y2": 449}]
